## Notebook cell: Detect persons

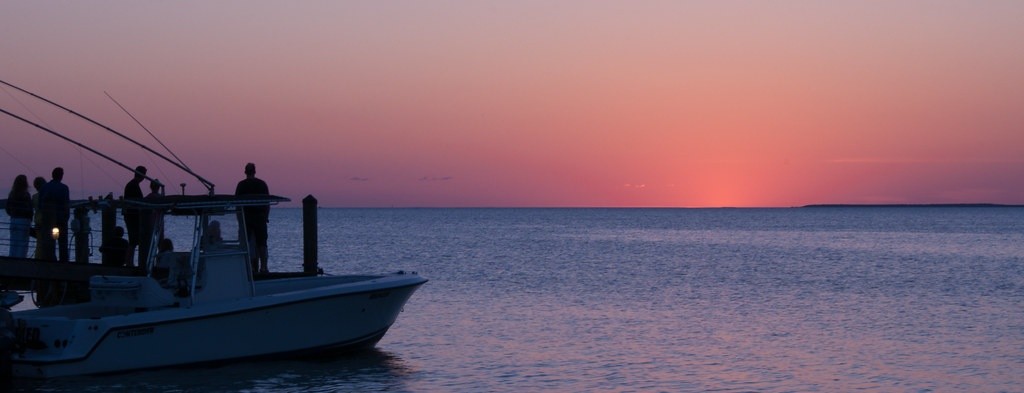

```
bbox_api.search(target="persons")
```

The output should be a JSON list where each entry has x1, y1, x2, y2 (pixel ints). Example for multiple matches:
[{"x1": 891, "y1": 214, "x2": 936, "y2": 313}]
[
  {"x1": 5, "y1": 163, "x2": 221, "y2": 268},
  {"x1": 235, "y1": 162, "x2": 270, "y2": 273}
]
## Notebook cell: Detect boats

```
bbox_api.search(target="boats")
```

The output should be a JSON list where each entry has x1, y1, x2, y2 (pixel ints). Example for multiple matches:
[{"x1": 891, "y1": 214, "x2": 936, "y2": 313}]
[{"x1": 0, "y1": 194, "x2": 429, "y2": 379}]
